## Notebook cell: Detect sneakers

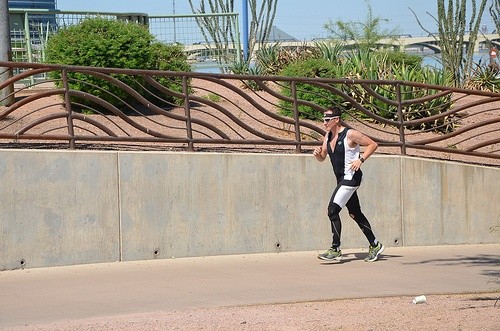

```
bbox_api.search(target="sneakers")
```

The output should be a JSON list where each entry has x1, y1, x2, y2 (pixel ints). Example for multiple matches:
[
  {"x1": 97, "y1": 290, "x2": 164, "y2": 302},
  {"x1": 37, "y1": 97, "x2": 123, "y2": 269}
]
[
  {"x1": 317, "y1": 248, "x2": 343, "y2": 261},
  {"x1": 365, "y1": 241, "x2": 386, "y2": 262}
]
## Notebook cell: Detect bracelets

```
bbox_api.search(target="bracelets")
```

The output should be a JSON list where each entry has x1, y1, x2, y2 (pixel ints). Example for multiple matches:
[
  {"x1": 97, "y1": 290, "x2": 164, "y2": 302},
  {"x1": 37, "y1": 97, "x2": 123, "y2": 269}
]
[{"x1": 359, "y1": 157, "x2": 364, "y2": 162}]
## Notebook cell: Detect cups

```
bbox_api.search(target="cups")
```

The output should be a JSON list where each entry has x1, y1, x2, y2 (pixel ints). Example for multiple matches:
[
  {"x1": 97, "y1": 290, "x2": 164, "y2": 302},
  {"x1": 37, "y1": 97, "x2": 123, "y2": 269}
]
[{"x1": 413, "y1": 295, "x2": 426, "y2": 304}]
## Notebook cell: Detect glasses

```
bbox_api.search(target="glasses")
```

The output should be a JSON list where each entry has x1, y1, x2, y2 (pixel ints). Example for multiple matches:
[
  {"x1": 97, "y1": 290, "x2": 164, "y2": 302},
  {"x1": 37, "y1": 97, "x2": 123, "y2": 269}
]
[{"x1": 321, "y1": 116, "x2": 340, "y2": 123}]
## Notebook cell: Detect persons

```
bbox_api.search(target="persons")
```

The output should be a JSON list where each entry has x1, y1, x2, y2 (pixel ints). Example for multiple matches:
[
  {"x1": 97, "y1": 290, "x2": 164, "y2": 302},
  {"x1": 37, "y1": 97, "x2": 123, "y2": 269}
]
[{"x1": 313, "y1": 107, "x2": 385, "y2": 263}]
